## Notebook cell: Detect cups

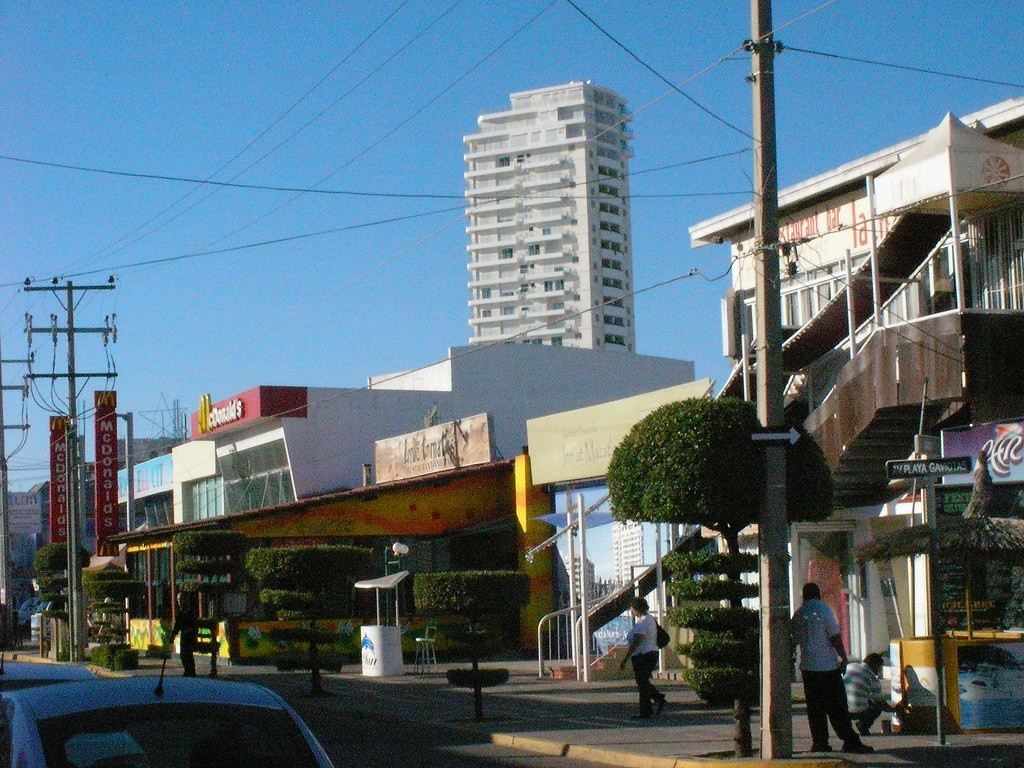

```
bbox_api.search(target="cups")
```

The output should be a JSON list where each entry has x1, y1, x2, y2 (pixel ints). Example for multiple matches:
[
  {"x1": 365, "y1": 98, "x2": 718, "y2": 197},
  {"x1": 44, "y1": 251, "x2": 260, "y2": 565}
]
[{"x1": 882, "y1": 720, "x2": 891, "y2": 733}]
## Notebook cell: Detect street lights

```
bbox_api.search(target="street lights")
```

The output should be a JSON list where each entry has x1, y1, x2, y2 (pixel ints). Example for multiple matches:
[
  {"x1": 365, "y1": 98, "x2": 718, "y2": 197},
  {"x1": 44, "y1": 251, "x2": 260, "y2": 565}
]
[{"x1": 384, "y1": 543, "x2": 408, "y2": 626}]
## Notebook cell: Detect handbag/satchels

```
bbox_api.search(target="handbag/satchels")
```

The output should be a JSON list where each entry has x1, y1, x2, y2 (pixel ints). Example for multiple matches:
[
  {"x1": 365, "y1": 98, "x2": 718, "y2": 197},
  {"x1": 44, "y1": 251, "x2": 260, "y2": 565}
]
[{"x1": 652, "y1": 617, "x2": 670, "y2": 649}]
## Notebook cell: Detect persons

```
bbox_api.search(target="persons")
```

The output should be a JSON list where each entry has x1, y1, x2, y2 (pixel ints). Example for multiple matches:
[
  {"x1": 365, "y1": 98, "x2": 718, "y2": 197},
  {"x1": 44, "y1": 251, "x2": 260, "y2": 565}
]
[
  {"x1": 841, "y1": 653, "x2": 896, "y2": 736},
  {"x1": 169, "y1": 592, "x2": 196, "y2": 678},
  {"x1": 620, "y1": 597, "x2": 668, "y2": 719},
  {"x1": 789, "y1": 582, "x2": 873, "y2": 754}
]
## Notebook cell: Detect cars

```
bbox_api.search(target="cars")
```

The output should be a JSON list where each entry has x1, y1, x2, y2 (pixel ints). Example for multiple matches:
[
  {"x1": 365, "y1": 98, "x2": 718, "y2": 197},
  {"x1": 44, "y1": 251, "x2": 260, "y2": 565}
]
[{"x1": 0, "y1": 659, "x2": 333, "y2": 768}]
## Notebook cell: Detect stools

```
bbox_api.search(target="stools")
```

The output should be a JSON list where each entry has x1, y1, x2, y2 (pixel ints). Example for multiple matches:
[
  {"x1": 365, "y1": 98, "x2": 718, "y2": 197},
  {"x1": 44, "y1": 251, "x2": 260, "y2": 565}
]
[{"x1": 413, "y1": 627, "x2": 438, "y2": 676}]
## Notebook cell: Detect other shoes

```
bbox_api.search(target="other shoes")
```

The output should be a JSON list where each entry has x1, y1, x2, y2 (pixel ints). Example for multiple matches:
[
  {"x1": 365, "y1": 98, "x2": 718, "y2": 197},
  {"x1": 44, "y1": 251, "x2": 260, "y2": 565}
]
[
  {"x1": 855, "y1": 720, "x2": 871, "y2": 736},
  {"x1": 839, "y1": 741, "x2": 874, "y2": 753},
  {"x1": 655, "y1": 693, "x2": 665, "y2": 717},
  {"x1": 811, "y1": 745, "x2": 832, "y2": 752}
]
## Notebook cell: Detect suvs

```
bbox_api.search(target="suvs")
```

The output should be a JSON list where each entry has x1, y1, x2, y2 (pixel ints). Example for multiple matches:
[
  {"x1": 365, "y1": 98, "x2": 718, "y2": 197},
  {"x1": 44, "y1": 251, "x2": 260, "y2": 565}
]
[{"x1": 20, "y1": 594, "x2": 101, "y2": 644}]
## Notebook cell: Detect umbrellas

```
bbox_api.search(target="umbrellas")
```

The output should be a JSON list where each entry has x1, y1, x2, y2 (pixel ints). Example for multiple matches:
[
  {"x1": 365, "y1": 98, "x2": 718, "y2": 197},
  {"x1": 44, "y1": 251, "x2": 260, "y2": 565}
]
[{"x1": 856, "y1": 514, "x2": 1024, "y2": 640}]
